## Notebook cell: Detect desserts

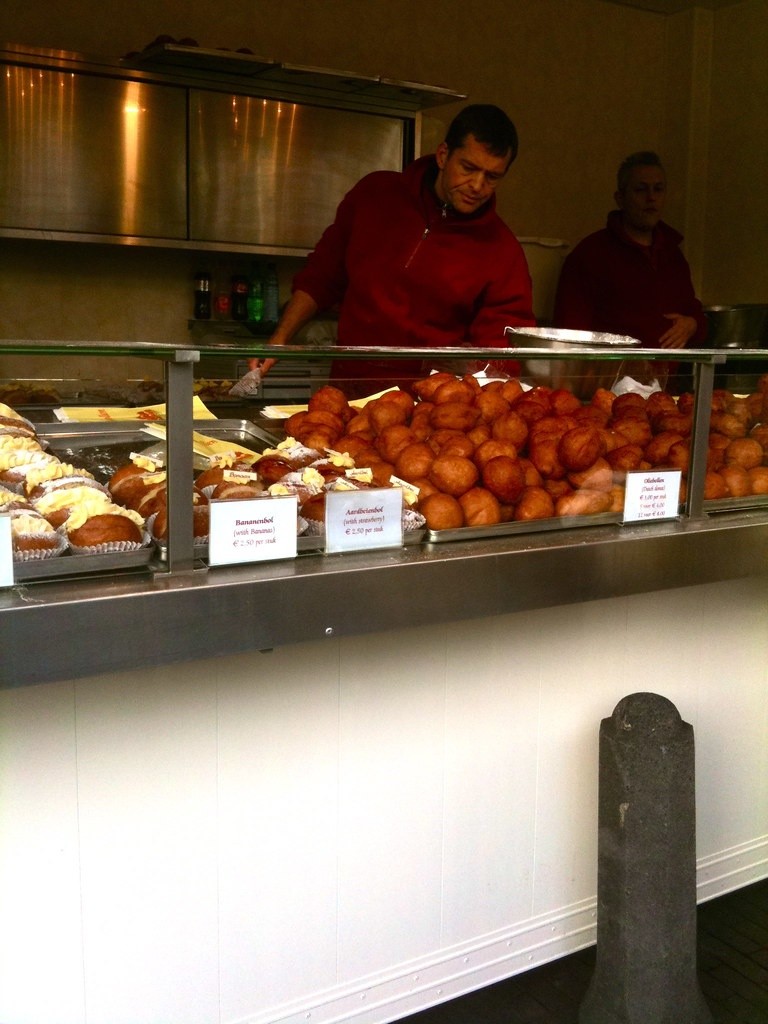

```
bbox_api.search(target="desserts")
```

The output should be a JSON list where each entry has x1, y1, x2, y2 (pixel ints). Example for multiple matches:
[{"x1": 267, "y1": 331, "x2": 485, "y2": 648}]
[{"x1": 1, "y1": 416, "x2": 390, "y2": 561}]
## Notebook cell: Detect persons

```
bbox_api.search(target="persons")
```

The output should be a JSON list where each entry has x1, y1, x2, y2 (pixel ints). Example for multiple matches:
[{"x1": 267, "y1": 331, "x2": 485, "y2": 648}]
[
  {"x1": 249, "y1": 105, "x2": 536, "y2": 402},
  {"x1": 555, "y1": 151, "x2": 709, "y2": 383}
]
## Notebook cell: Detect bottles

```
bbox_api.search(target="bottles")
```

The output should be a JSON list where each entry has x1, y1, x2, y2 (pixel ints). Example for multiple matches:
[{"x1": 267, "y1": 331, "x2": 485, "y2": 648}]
[
  {"x1": 194, "y1": 272, "x2": 231, "y2": 320},
  {"x1": 232, "y1": 269, "x2": 250, "y2": 322},
  {"x1": 263, "y1": 273, "x2": 281, "y2": 323},
  {"x1": 246, "y1": 270, "x2": 265, "y2": 322}
]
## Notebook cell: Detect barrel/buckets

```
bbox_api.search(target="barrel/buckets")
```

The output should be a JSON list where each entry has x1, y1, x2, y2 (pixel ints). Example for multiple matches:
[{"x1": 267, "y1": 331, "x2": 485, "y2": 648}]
[{"x1": 705, "y1": 303, "x2": 768, "y2": 361}]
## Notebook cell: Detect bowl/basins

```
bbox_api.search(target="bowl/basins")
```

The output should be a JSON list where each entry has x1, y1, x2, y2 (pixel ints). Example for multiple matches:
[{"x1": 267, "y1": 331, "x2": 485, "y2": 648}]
[{"x1": 507, "y1": 326, "x2": 642, "y2": 403}]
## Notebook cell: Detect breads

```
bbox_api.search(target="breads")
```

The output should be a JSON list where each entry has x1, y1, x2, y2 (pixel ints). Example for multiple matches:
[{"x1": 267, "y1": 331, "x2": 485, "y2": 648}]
[{"x1": 283, "y1": 372, "x2": 768, "y2": 531}]
